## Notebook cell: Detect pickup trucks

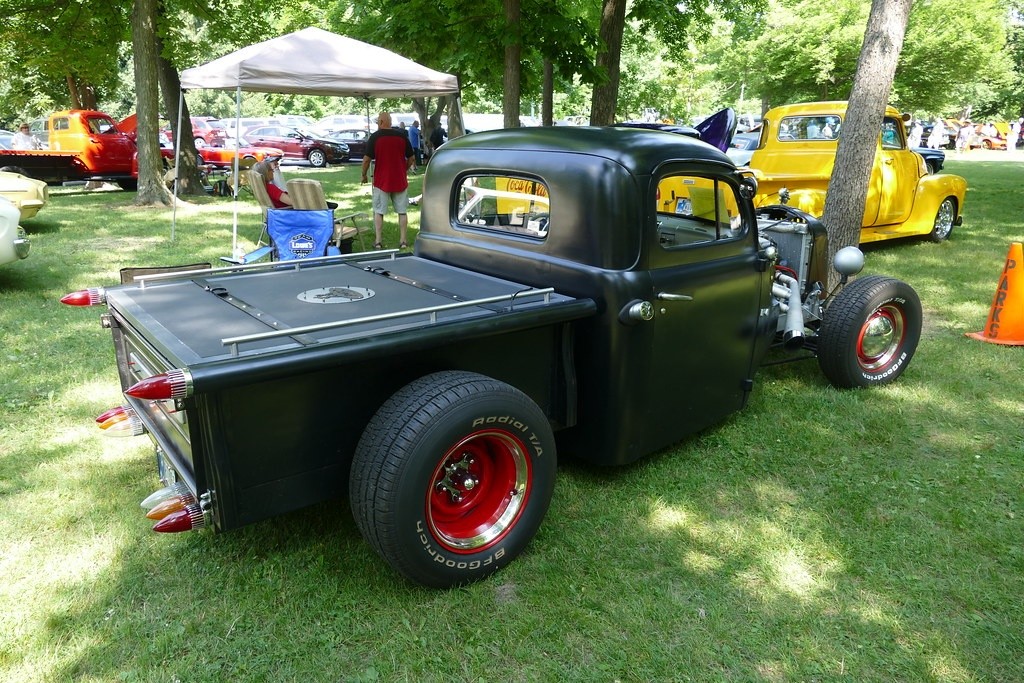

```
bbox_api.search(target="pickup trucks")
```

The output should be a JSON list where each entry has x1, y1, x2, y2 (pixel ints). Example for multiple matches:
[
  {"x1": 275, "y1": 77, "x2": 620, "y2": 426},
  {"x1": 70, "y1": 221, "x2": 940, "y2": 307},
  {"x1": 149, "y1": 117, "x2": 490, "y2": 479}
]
[
  {"x1": 60, "y1": 125, "x2": 924, "y2": 593},
  {"x1": 494, "y1": 99, "x2": 969, "y2": 246},
  {"x1": 0, "y1": 110, "x2": 172, "y2": 192}
]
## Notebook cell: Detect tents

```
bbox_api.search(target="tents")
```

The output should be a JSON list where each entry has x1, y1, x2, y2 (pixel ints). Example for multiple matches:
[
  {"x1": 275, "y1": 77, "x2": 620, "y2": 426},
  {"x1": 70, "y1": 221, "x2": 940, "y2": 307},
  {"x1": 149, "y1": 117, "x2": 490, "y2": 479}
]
[{"x1": 171, "y1": 26, "x2": 466, "y2": 263}]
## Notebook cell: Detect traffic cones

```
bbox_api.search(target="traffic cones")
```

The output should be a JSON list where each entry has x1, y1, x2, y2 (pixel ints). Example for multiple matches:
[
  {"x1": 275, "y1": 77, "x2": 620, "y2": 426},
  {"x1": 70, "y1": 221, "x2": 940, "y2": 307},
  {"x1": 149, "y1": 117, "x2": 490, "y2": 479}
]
[{"x1": 963, "y1": 242, "x2": 1024, "y2": 346}]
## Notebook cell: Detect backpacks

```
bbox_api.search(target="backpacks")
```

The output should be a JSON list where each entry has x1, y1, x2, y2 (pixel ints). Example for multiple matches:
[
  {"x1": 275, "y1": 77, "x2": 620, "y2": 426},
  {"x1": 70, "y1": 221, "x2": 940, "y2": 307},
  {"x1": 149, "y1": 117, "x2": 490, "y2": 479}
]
[{"x1": 213, "y1": 180, "x2": 231, "y2": 197}]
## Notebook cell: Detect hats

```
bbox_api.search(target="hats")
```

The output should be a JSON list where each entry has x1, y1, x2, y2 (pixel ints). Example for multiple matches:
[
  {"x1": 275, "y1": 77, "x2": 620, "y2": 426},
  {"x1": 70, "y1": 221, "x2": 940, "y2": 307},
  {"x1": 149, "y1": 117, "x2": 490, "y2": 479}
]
[
  {"x1": 914, "y1": 120, "x2": 921, "y2": 125},
  {"x1": 264, "y1": 157, "x2": 280, "y2": 163},
  {"x1": 18, "y1": 123, "x2": 30, "y2": 131},
  {"x1": 827, "y1": 118, "x2": 836, "y2": 126},
  {"x1": 963, "y1": 119, "x2": 971, "y2": 125}
]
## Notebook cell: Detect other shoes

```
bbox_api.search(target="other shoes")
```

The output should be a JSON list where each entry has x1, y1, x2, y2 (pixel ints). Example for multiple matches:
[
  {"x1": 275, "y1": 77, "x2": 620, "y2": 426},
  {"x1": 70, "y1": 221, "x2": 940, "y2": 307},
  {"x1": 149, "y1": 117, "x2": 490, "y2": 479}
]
[{"x1": 326, "y1": 201, "x2": 338, "y2": 209}]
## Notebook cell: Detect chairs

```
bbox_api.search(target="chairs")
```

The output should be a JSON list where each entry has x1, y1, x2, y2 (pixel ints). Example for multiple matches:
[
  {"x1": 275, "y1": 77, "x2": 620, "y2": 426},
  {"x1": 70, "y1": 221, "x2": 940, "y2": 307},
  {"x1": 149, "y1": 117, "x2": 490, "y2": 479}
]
[{"x1": 116, "y1": 157, "x2": 366, "y2": 285}]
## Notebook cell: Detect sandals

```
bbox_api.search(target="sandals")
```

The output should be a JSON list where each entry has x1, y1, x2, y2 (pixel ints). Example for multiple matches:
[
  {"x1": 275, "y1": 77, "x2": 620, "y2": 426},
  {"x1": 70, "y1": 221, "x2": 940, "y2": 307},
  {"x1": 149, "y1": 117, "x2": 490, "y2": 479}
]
[
  {"x1": 372, "y1": 242, "x2": 382, "y2": 249},
  {"x1": 400, "y1": 242, "x2": 407, "y2": 249}
]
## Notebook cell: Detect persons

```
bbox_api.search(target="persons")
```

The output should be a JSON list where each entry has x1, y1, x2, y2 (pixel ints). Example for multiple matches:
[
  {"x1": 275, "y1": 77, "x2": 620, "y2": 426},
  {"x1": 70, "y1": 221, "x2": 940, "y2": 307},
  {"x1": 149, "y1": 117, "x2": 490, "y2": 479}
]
[
  {"x1": 253, "y1": 154, "x2": 338, "y2": 211},
  {"x1": 906, "y1": 117, "x2": 1023, "y2": 154},
  {"x1": 656, "y1": 113, "x2": 676, "y2": 125},
  {"x1": 361, "y1": 112, "x2": 414, "y2": 250},
  {"x1": 11, "y1": 123, "x2": 43, "y2": 151},
  {"x1": 398, "y1": 118, "x2": 449, "y2": 175},
  {"x1": 781, "y1": 119, "x2": 833, "y2": 139}
]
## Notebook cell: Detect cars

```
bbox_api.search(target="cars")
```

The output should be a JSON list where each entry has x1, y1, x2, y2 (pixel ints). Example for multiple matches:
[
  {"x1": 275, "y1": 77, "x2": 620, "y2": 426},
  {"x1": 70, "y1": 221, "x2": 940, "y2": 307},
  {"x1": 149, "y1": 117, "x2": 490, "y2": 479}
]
[
  {"x1": 0, "y1": 115, "x2": 49, "y2": 151},
  {"x1": 724, "y1": 105, "x2": 1024, "y2": 169},
  {"x1": 117, "y1": 112, "x2": 474, "y2": 175}
]
